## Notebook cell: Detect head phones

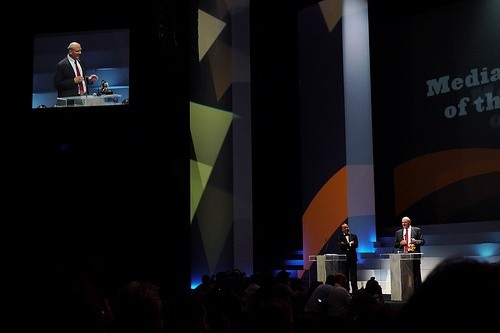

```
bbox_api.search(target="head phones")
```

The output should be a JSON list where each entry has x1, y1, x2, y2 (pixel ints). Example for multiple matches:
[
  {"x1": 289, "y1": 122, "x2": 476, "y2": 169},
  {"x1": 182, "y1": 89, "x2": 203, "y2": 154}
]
[{"x1": 230, "y1": 268, "x2": 244, "y2": 278}]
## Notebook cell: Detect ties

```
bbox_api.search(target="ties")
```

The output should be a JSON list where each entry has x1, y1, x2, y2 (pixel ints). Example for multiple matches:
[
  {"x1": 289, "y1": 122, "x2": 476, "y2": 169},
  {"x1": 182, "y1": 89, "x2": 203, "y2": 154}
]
[
  {"x1": 75, "y1": 61, "x2": 84, "y2": 96},
  {"x1": 404, "y1": 229, "x2": 408, "y2": 253}
]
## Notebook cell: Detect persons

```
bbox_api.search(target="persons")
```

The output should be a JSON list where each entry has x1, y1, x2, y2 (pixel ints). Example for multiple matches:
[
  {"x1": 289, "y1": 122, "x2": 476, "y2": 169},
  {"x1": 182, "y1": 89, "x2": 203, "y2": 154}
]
[
  {"x1": 1, "y1": 257, "x2": 499, "y2": 332},
  {"x1": 98, "y1": 79, "x2": 113, "y2": 97},
  {"x1": 339, "y1": 223, "x2": 360, "y2": 291},
  {"x1": 54, "y1": 41, "x2": 99, "y2": 106},
  {"x1": 396, "y1": 216, "x2": 427, "y2": 294}
]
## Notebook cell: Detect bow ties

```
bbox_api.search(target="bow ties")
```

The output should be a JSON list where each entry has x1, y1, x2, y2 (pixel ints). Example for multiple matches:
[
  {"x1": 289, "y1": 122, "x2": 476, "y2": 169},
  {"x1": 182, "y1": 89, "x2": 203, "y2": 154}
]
[{"x1": 344, "y1": 233, "x2": 350, "y2": 236}]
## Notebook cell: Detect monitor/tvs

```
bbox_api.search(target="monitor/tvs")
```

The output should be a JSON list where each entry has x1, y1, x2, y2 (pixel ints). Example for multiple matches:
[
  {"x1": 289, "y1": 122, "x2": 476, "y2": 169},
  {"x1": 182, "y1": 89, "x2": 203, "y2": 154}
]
[{"x1": 26, "y1": 25, "x2": 134, "y2": 116}]
